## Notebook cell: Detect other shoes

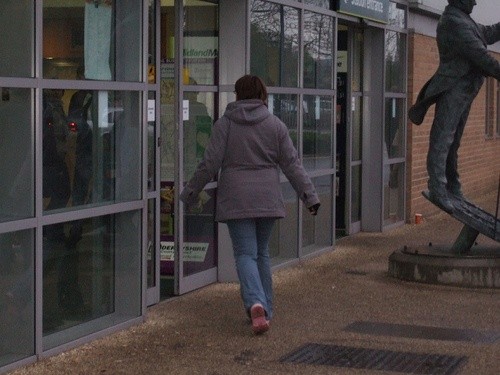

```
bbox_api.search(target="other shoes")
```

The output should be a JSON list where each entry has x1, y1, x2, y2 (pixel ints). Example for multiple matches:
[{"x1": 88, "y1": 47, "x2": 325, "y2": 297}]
[{"x1": 250, "y1": 303, "x2": 268, "y2": 334}]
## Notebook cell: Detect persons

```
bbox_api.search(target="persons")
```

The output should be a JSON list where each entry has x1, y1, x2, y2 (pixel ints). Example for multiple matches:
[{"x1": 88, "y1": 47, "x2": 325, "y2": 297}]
[
  {"x1": 406, "y1": 0, "x2": 500, "y2": 213},
  {"x1": 179, "y1": 73, "x2": 321, "y2": 335}
]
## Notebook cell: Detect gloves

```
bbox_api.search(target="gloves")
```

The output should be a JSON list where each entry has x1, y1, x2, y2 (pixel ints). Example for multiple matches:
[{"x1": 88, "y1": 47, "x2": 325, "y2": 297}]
[{"x1": 309, "y1": 202, "x2": 320, "y2": 215}]
